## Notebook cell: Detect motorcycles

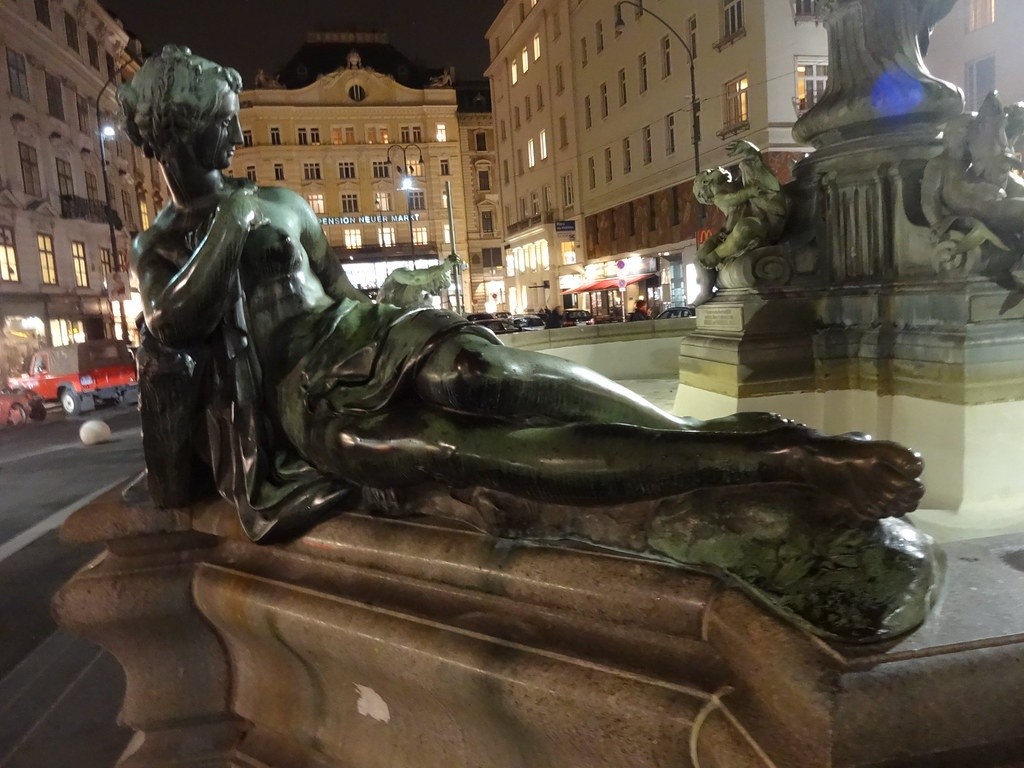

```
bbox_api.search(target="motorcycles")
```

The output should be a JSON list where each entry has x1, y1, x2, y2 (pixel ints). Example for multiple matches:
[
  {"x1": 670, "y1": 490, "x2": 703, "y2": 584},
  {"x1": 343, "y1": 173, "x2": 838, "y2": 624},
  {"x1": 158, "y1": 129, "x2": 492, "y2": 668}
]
[{"x1": 1, "y1": 382, "x2": 47, "y2": 421}]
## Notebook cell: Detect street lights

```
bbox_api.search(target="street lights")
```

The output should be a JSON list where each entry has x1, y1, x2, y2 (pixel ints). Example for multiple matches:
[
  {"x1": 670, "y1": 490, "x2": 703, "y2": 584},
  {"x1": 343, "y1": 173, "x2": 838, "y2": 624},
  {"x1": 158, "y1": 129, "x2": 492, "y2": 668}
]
[
  {"x1": 94, "y1": 49, "x2": 157, "y2": 343},
  {"x1": 614, "y1": 0, "x2": 699, "y2": 178},
  {"x1": 385, "y1": 143, "x2": 424, "y2": 270}
]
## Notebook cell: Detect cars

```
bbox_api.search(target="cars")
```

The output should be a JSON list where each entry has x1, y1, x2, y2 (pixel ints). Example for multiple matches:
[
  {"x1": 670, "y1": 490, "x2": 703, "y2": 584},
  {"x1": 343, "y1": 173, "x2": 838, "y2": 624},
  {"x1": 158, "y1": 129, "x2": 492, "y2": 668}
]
[
  {"x1": 0, "y1": 384, "x2": 31, "y2": 430},
  {"x1": 560, "y1": 309, "x2": 595, "y2": 327},
  {"x1": 655, "y1": 307, "x2": 695, "y2": 319},
  {"x1": 463, "y1": 312, "x2": 549, "y2": 334}
]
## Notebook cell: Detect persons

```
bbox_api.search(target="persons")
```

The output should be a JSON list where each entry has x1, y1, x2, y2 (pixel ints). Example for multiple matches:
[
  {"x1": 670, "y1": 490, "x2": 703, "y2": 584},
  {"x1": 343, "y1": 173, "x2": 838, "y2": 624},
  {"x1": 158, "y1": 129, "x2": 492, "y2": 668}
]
[
  {"x1": 921, "y1": 88, "x2": 1024, "y2": 226},
  {"x1": 544, "y1": 306, "x2": 567, "y2": 329},
  {"x1": 632, "y1": 299, "x2": 652, "y2": 322},
  {"x1": 114, "y1": 48, "x2": 927, "y2": 542},
  {"x1": 544, "y1": 306, "x2": 549, "y2": 313}
]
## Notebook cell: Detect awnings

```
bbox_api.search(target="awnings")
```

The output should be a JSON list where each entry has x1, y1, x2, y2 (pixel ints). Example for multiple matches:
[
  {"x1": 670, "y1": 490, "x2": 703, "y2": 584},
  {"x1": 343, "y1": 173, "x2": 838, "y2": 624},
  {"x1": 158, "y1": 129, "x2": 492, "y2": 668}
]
[{"x1": 560, "y1": 274, "x2": 655, "y2": 295}]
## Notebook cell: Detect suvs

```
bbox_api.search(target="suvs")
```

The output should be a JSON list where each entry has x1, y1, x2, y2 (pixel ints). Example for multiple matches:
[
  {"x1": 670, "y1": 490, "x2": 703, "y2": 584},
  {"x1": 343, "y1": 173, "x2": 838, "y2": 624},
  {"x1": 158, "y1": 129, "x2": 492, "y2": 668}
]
[{"x1": 6, "y1": 339, "x2": 141, "y2": 416}]
